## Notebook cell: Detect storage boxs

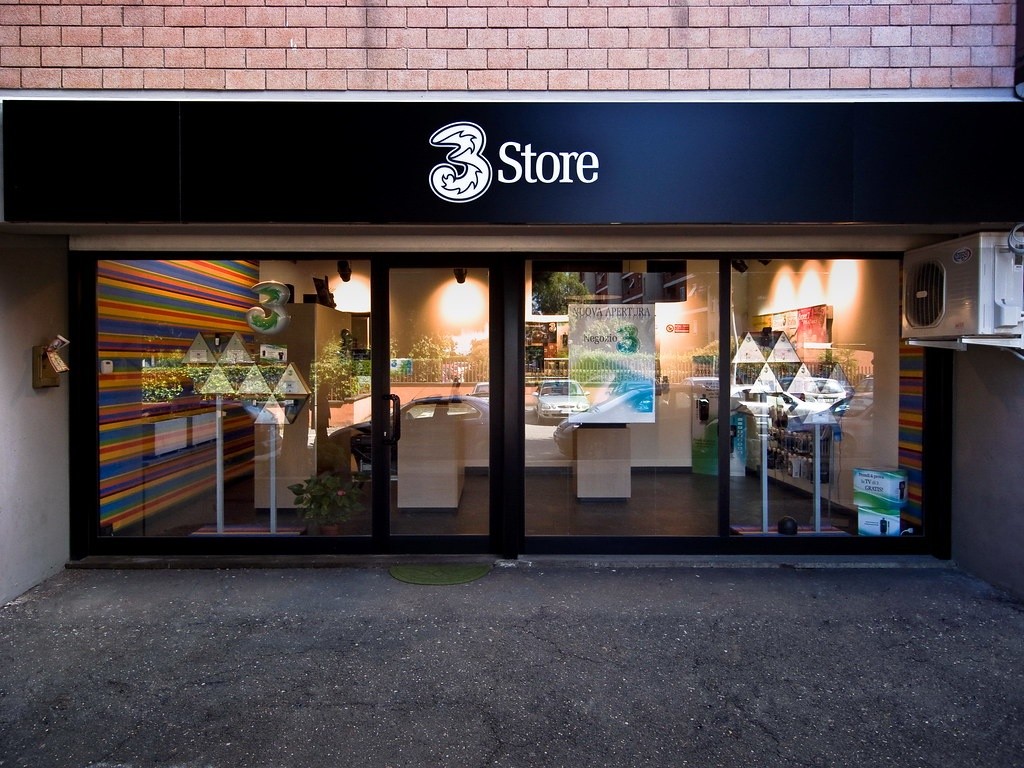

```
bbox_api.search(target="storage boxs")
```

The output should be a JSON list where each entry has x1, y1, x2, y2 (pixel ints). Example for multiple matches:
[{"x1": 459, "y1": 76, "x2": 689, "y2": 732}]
[
  {"x1": 851, "y1": 467, "x2": 909, "y2": 509},
  {"x1": 857, "y1": 506, "x2": 901, "y2": 535}
]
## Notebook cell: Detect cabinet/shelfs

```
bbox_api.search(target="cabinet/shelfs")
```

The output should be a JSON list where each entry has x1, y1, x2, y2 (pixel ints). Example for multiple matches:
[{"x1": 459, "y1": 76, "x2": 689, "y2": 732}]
[
  {"x1": 745, "y1": 259, "x2": 900, "y2": 513},
  {"x1": 256, "y1": 304, "x2": 370, "y2": 509}
]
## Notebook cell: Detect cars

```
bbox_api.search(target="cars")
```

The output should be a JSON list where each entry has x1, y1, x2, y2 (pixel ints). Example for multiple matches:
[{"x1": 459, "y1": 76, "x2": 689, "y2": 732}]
[
  {"x1": 681, "y1": 377, "x2": 720, "y2": 388},
  {"x1": 851, "y1": 377, "x2": 872, "y2": 393},
  {"x1": 789, "y1": 393, "x2": 873, "y2": 454},
  {"x1": 533, "y1": 379, "x2": 590, "y2": 425},
  {"x1": 466, "y1": 382, "x2": 489, "y2": 405},
  {"x1": 325, "y1": 395, "x2": 491, "y2": 478},
  {"x1": 777, "y1": 378, "x2": 846, "y2": 404},
  {"x1": 605, "y1": 382, "x2": 654, "y2": 399},
  {"x1": 553, "y1": 384, "x2": 759, "y2": 460},
  {"x1": 730, "y1": 386, "x2": 840, "y2": 432}
]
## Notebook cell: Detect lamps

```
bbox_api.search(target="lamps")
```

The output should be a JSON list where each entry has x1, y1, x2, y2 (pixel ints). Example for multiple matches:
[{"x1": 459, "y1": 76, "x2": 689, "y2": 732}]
[
  {"x1": 337, "y1": 260, "x2": 352, "y2": 283},
  {"x1": 731, "y1": 259, "x2": 748, "y2": 273},
  {"x1": 453, "y1": 268, "x2": 467, "y2": 283}
]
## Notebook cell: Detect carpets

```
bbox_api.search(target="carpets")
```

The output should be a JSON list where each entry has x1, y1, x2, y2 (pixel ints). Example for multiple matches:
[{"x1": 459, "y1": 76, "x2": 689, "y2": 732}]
[{"x1": 388, "y1": 563, "x2": 492, "y2": 585}]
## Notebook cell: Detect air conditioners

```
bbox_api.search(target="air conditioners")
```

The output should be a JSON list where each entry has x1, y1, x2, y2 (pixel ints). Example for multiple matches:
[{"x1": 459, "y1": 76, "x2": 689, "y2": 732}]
[{"x1": 901, "y1": 232, "x2": 1024, "y2": 340}]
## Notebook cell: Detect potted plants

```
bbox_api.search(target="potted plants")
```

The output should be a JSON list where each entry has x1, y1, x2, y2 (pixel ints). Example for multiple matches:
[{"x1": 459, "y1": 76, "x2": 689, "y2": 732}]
[{"x1": 289, "y1": 473, "x2": 368, "y2": 536}]
